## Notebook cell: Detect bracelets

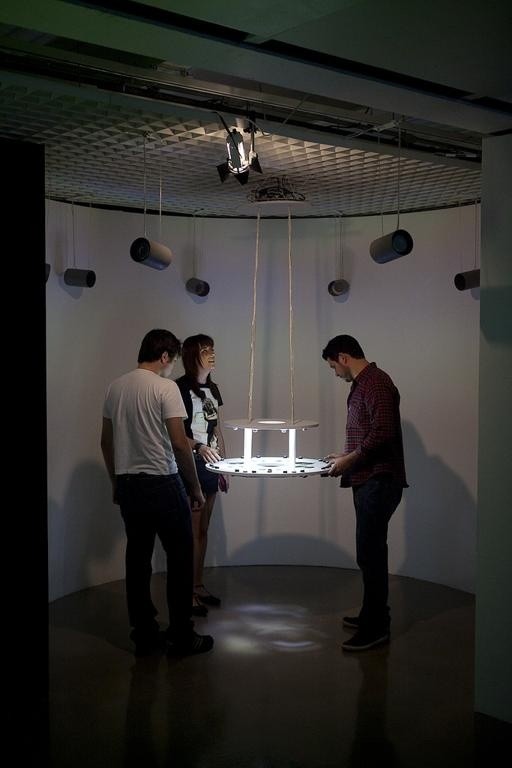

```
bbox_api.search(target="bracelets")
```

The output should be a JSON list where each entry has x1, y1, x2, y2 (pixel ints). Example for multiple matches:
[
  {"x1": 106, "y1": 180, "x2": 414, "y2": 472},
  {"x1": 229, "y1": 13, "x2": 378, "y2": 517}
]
[{"x1": 194, "y1": 442, "x2": 204, "y2": 453}]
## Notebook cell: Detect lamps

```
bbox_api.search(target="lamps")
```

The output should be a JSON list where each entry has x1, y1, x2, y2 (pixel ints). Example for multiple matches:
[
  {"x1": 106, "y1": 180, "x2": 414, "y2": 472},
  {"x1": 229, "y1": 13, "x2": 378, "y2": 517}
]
[
  {"x1": 62, "y1": 197, "x2": 97, "y2": 287},
  {"x1": 328, "y1": 211, "x2": 350, "y2": 299},
  {"x1": 203, "y1": 179, "x2": 336, "y2": 479},
  {"x1": 134, "y1": 133, "x2": 173, "y2": 273},
  {"x1": 209, "y1": 110, "x2": 264, "y2": 188},
  {"x1": 365, "y1": 116, "x2": 414, "y2": 264},
  {"x1": 451, "y1": 194, "x2": 480, "y2": 292},
  {"x1": 186, "y1": 211, "x2": 211, "y2": 298}
]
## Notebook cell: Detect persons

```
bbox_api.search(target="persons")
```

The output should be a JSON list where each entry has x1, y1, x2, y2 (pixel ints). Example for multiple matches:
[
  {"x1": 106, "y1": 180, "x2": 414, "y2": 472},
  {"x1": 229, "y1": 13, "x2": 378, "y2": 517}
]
[
  {"x1": 173, "y1": 332, "x2": 230, "y2": 618},
  {"x1": 316, "y1": 331, "x2": 410, "y2": 653},
  {"x1": 99, "y1": 328, "x2": 215, "y2": 659}
]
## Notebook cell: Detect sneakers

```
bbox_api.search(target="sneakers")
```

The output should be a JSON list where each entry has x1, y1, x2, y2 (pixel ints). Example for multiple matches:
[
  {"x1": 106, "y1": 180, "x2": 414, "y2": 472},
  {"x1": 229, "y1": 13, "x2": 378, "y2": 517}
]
[
  {"x1": 134, "y1": 629, "x2": 164, "y2": 651},
  {"x1": 342, "y1": 615, "x2": 359, "y2": 627},
  {"x1": 163, "y1": 630, "x2": 214, "y2": 660},
  {"x1": 341, "y1": 622, "x2": 391, "y2": 652}
]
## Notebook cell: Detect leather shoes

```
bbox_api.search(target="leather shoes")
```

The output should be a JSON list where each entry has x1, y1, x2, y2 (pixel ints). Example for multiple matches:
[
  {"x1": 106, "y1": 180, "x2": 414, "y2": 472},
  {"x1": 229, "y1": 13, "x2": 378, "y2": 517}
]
[
  {"x1": 194, "y1": 584, "x2": 221, "y2": 608},
  {"x1": 191, "y1": 597, "x2": 210, "y2": 618}
]
